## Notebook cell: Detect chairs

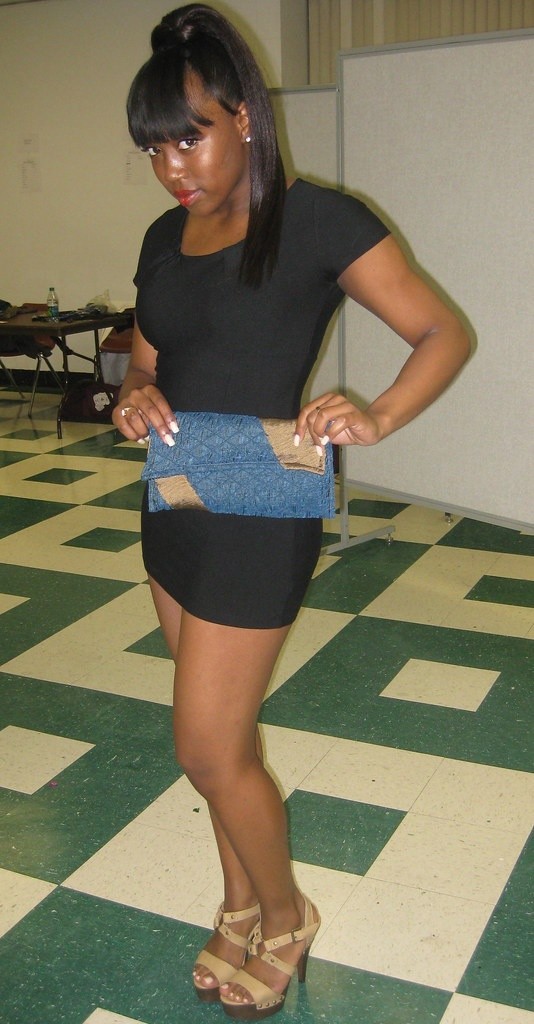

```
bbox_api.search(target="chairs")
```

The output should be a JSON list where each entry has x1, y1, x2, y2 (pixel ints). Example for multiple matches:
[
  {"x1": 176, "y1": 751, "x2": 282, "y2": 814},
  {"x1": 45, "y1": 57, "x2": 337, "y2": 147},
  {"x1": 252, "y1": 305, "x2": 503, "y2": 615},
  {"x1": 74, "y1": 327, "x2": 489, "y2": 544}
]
[{"x1": 0, "y1": 303, "x2": 64, "y2": 420}]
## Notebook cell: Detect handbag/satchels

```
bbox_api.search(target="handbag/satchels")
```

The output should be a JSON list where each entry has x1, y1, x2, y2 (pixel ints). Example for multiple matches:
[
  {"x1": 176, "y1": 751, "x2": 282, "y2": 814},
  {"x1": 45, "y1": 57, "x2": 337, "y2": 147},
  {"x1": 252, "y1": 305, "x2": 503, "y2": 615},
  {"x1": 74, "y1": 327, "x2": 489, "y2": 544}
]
[{"x1": 144, "y1": 409, "x2": 337, "y2": 521}]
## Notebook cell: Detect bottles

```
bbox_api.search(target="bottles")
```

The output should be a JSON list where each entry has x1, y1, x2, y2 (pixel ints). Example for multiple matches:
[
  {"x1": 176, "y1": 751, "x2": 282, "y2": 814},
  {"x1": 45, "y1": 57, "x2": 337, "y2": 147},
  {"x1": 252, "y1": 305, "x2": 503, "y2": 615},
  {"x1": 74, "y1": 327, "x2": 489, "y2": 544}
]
[{"x1": 46, "y1": 287, "x2": 59, "y2": 326}]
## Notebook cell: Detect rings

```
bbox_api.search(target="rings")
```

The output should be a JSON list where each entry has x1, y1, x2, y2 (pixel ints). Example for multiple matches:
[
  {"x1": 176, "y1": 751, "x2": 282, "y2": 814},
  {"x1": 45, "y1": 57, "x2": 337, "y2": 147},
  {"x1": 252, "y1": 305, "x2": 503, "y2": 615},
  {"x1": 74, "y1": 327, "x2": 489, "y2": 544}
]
[
  {"x1": 316, "y1": 406, "x2": 321, "y2": 413},
  {"x1": 122, "y1": 406, "x2": 137, "y2": 417}
]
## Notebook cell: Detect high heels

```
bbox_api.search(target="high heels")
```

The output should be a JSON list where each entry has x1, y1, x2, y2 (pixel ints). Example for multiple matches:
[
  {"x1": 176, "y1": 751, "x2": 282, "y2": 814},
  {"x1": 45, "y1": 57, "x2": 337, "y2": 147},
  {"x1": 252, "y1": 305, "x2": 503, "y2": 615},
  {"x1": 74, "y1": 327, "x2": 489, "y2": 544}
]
[{"x1": 191, "y1": 893, "x2": 321, "y2": 1022}]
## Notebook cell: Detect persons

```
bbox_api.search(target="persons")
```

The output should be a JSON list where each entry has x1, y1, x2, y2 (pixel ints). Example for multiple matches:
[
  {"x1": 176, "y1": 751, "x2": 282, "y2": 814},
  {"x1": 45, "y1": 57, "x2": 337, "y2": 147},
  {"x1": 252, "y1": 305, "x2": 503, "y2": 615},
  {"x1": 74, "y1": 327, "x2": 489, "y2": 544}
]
[{"x1": 112, "y1": 3, "x2": 471, "y2": 1022}]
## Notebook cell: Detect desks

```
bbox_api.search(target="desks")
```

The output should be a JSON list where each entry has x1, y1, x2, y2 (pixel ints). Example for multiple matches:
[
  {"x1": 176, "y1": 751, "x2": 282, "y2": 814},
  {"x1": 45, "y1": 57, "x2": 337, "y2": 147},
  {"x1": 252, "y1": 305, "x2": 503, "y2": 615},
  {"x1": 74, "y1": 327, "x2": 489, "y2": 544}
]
[{"x1": 0, "y1": 311, "x2": 131, "y2": 389}]
